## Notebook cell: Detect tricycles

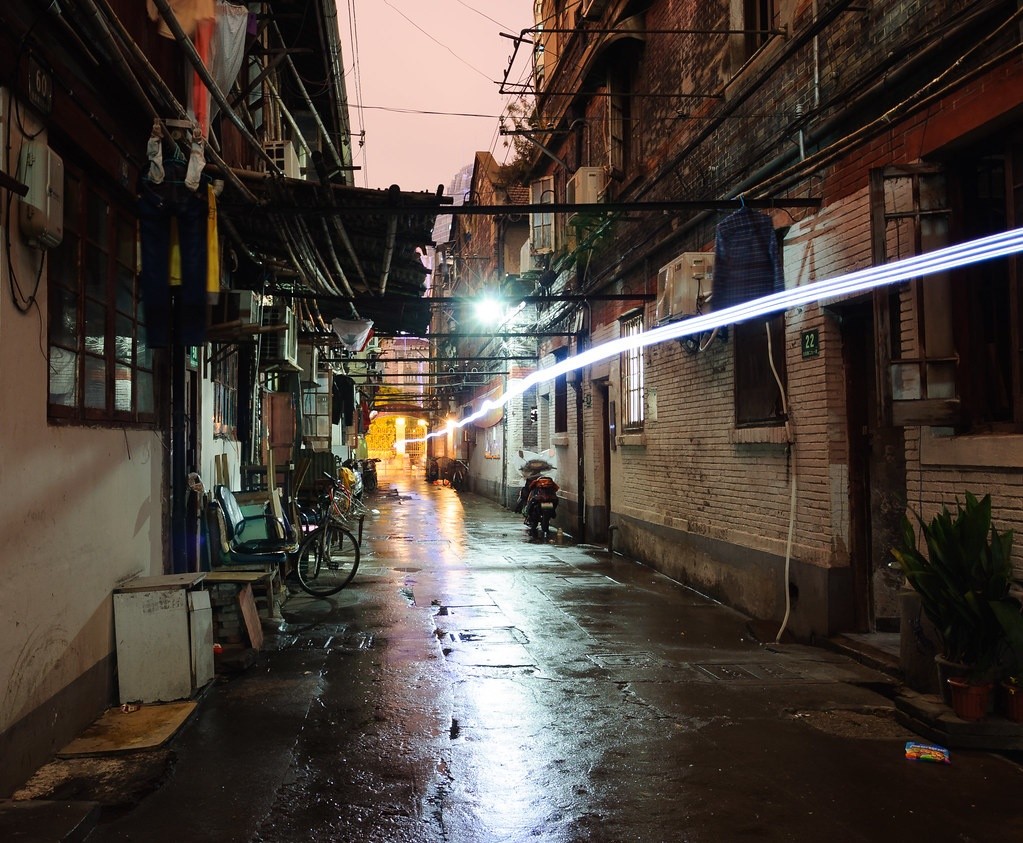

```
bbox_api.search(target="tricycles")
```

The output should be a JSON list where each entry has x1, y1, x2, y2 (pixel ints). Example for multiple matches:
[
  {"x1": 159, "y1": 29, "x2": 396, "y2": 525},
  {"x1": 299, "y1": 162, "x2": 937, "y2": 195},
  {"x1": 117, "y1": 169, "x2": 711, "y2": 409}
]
[{"x1": 515, "y1": 449, "x2": 556, "y2": 513}]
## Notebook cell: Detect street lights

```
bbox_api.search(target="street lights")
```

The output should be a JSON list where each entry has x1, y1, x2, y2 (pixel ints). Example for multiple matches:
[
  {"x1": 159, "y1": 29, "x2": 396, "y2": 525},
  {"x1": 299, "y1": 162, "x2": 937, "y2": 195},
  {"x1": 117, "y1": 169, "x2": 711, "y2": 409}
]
[{"x1": 475, "y1": 296, "x2": 593, "y2": 362}]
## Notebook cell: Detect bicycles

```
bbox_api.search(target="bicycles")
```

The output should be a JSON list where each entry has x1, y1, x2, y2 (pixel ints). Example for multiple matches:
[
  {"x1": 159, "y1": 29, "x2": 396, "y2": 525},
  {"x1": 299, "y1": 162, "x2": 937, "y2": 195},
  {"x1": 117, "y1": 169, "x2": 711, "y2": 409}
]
[
  {"x1": 331, "y1": 454, "x2": 353, "y2": 513},
  {"x1": 443, "y1": 456, "x2": 463, "y2": 490},
  {"x1": 294, "y1": 471, "x2": 372, "y2": 596}
]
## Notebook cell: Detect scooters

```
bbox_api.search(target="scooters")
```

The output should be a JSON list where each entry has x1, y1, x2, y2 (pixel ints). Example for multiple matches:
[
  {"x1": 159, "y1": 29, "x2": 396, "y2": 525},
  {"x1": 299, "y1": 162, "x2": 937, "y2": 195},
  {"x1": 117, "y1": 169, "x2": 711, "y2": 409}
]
[
  {"x1": 427, "y1": 457, "x2": 441, "y2": 481},
  {"x1": 518, "y1": 450, "x2": 560, "y2": 536},
  {"x1": 342, "y1": 454, "x2": 364, "y2": 511},
  {"x1": 358, "y1": 457, "x2": 381, "y2": 493}
]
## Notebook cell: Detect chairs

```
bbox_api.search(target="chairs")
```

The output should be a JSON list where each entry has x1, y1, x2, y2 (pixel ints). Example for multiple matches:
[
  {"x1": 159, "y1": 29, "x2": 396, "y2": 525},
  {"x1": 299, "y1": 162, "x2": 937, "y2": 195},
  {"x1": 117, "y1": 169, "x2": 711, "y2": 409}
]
[
  {"x1": 208, "y1": 501, "x2": 281, "y2": 618},
  {"x1": 217, "y1": 485, "x2": 300, "y2": 589}
]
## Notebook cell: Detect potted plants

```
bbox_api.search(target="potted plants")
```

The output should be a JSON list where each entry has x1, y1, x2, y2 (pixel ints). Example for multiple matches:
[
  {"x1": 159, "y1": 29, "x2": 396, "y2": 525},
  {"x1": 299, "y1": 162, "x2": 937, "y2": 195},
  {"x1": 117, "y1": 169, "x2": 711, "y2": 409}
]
[
  {"x1": 892, "y1": 490, "x2": 1013, "y2": 710},
  {"x1": 948, "y1": 565, "x2": 991, "y2": 721},
  {"x1": 991, "y1": 600, "x2": 1023, "y2": 722}
]
